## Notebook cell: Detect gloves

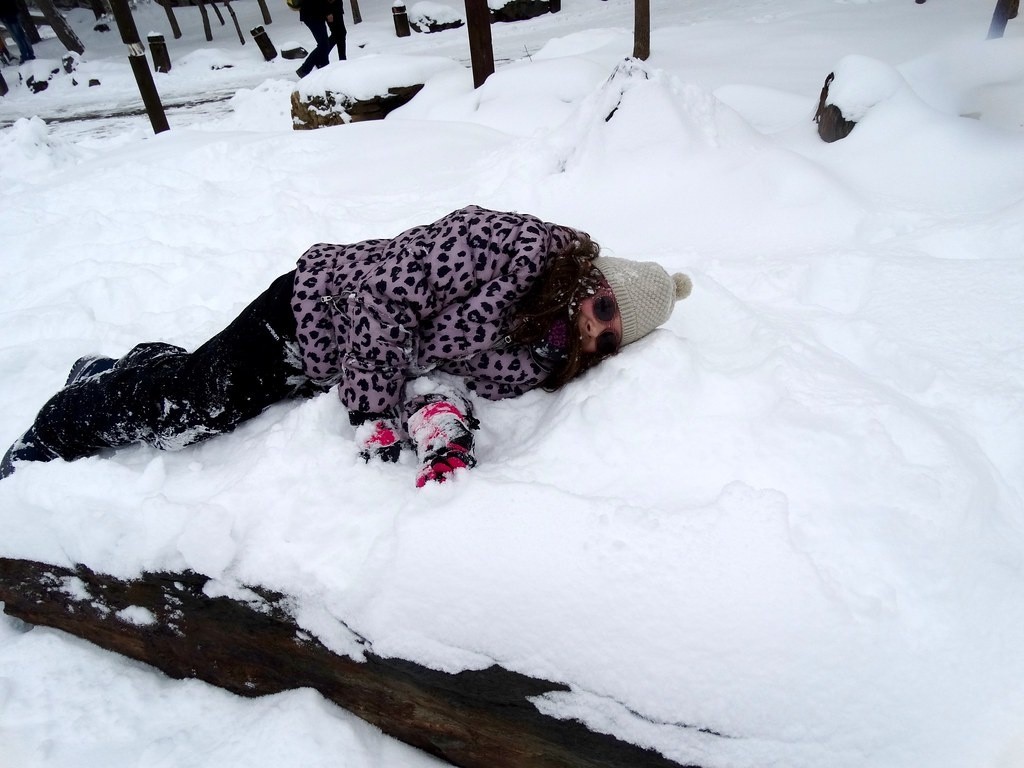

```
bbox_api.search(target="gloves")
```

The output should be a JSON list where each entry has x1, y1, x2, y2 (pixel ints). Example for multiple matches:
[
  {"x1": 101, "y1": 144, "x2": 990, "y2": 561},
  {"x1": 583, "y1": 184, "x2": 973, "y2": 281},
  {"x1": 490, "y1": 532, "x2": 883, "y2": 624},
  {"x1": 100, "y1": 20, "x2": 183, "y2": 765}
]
[
  {"x1": 408, "y1": 401, "x2": 477, "y2": 488},
  {"x1": 357, "y1": 419, "x2": 405, "y2": 464}
]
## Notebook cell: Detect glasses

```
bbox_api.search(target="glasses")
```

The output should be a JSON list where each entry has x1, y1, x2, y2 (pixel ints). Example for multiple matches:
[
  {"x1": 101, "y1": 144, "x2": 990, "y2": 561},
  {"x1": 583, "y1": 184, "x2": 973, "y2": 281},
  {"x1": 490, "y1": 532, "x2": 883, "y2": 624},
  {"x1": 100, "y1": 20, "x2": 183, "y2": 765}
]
[{"x1": 592, "y1": 289, "x2": 620, "y2": 355}]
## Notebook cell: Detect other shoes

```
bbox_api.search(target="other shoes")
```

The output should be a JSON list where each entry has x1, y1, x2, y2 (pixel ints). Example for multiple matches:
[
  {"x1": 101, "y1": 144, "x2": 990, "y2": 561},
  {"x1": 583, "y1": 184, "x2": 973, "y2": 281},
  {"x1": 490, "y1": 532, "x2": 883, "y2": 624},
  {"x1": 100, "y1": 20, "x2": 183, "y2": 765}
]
[
  {"x1": 0, "y1": 425, "x2": 59, "y2": 480},
  {"x1": 65, "y1": 355, "x2": 119, "y2": 387}
]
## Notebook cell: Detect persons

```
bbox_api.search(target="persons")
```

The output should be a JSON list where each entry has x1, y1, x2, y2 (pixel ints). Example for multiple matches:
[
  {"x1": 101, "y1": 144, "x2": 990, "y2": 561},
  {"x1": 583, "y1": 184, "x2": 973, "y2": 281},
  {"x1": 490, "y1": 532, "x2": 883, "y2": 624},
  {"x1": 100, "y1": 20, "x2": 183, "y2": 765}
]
[
  {"x1": 1, "y1": 204, "x2": 691, "y2": 487},
  {"x1": 287, "y1": 0, "x2": 347, "y2": 79}
]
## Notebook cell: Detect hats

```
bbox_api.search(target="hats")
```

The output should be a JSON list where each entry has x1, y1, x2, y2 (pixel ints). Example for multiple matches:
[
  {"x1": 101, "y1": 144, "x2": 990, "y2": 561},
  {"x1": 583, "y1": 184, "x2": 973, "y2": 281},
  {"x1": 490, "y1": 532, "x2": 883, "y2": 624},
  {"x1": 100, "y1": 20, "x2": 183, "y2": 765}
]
[{"x1": 592, "y1": 256, "x2": 692, "y2": 347}]
